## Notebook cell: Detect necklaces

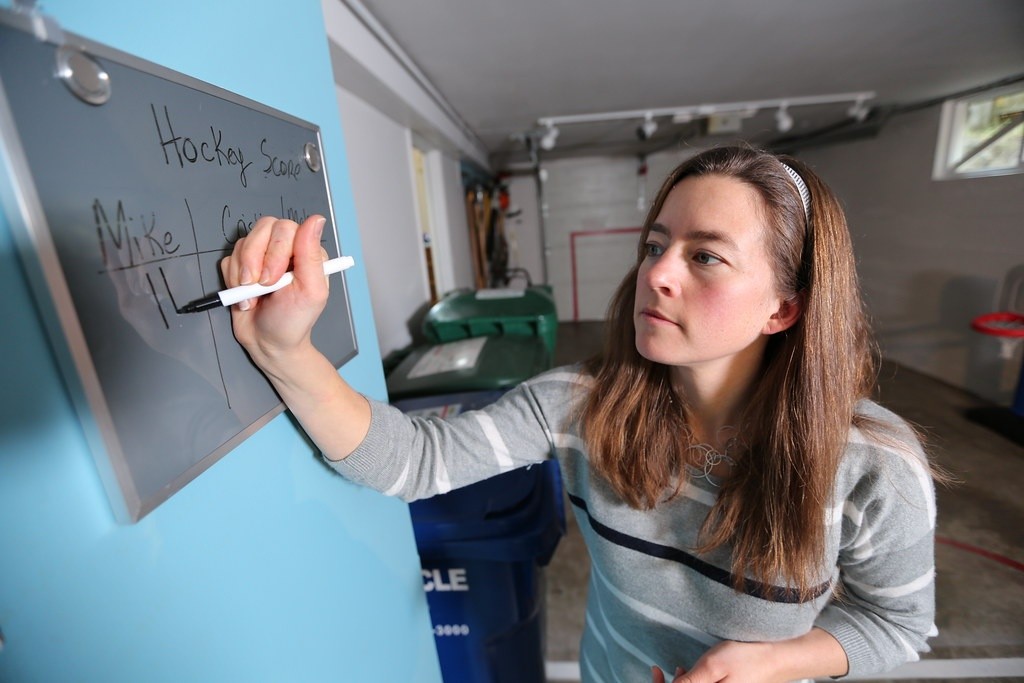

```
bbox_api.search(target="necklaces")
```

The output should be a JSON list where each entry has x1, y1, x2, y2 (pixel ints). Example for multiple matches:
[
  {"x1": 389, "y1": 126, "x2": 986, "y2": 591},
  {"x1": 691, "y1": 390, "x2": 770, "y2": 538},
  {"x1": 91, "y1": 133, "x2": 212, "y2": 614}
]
[{"x1": 672, "y1": 421, "x2": 744, "y2": 489}]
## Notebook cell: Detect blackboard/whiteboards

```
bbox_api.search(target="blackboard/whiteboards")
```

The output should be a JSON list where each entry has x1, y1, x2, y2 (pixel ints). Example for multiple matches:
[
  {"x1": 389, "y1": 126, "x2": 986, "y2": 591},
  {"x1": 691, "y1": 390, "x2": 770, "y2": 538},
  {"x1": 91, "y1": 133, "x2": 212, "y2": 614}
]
[{"x1": 1, "y1": 14, "x2": 360, "y2": 528}]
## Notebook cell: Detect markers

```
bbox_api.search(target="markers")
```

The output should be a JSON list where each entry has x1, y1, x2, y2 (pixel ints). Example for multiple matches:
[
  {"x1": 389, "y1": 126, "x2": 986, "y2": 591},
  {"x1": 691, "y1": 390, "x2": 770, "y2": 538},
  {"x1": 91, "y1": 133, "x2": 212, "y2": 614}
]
[{"x1": 176, "y1": 254, "x2": 356, "y2": 318}]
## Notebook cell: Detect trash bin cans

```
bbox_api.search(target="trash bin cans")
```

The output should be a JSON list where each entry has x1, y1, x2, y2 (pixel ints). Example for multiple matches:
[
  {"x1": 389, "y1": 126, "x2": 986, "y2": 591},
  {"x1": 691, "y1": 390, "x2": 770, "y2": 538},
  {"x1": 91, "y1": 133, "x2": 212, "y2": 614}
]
[{"x1": 390, "y1": 284, "x2": 560, "y2": 683}]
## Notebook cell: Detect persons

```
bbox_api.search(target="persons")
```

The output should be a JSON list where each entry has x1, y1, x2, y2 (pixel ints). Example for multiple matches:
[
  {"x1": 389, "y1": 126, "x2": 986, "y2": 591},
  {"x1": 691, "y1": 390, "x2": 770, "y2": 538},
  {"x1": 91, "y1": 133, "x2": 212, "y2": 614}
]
[{"x1": 220, "y1": 146, "x2": 941, "y2": 682}]
